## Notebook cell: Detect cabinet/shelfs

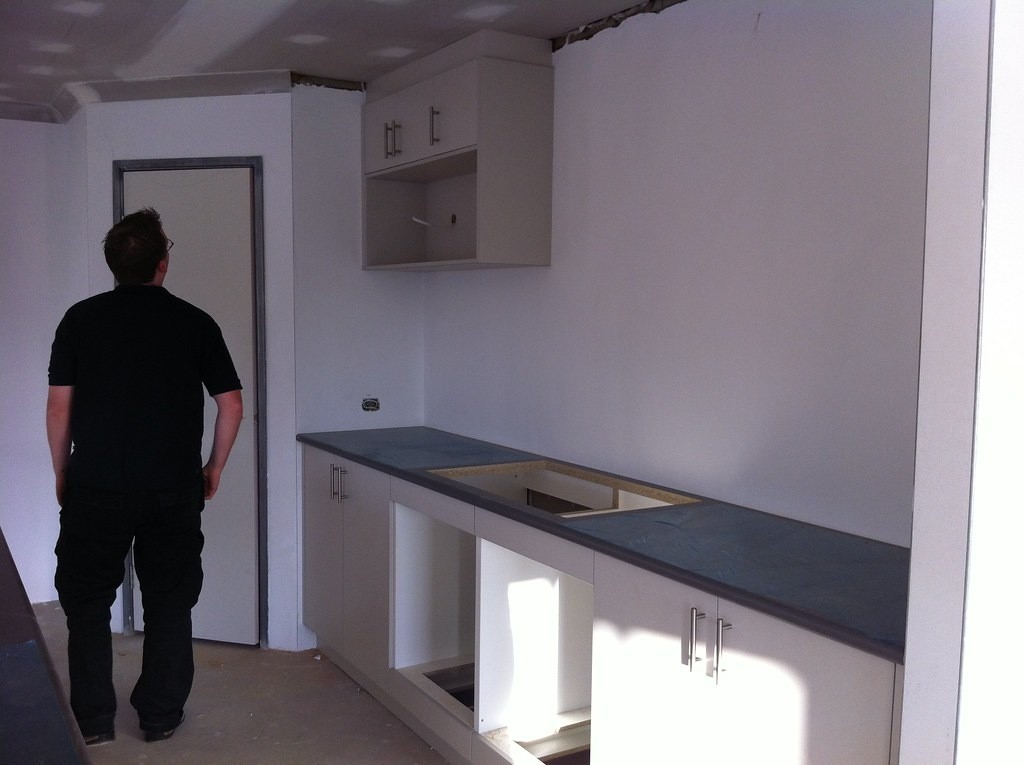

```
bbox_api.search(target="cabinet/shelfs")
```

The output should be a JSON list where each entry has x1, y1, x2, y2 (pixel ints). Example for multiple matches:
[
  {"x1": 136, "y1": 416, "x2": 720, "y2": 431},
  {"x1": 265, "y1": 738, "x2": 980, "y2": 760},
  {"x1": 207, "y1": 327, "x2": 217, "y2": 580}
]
[
  {"x1": 294, "y1": 425, "x2": 527, "y2": 688},
  {"x1": 588, "y1": 514, "x2": 901, "y2": 763},
  {"x1": 361, "y1": 31, "x2": 555, "y2": 274}
]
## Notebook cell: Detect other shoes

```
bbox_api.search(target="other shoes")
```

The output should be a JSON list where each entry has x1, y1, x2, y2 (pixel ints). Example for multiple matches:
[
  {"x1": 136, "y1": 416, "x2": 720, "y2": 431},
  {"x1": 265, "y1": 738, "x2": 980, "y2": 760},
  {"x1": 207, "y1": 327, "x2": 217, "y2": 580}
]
[
  {"x1": 80, "y1": 730, "x2": 116, "y2": 745},
  {"x1": 143, "y1": 722, "x2": 174, "y2": 742}
]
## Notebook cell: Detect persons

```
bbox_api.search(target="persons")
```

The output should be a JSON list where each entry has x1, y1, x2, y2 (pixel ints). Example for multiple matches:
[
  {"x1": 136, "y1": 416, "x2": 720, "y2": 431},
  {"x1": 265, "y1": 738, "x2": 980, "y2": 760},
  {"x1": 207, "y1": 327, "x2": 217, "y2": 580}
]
[{"x1": 44, "y1": 204, "x2": 247, "y2": 744}]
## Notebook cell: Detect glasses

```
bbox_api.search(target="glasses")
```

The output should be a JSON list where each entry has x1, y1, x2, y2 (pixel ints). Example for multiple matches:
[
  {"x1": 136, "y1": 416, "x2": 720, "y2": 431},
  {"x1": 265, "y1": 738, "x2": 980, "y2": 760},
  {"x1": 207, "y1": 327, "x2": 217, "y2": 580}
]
[{"x1": 165, "y1": 239, "x2": 173, "y2": 252}]
